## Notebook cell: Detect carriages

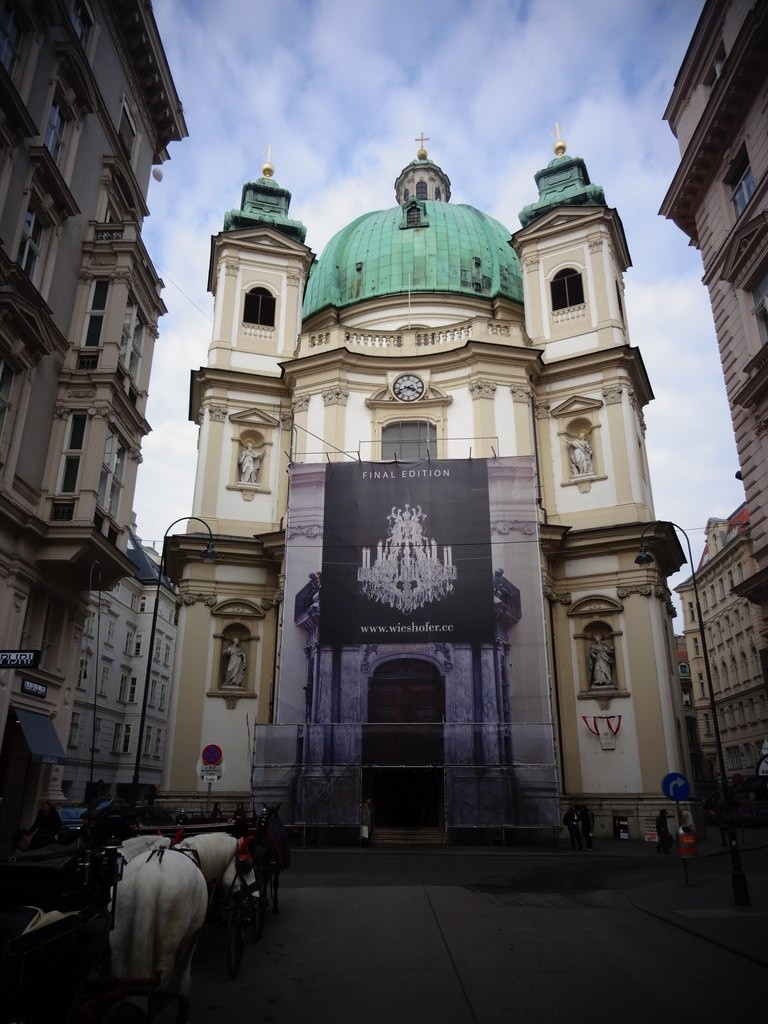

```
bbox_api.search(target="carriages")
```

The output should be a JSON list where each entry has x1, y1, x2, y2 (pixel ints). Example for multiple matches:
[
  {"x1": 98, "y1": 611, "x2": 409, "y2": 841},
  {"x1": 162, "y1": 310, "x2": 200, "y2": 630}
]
[
  {"x1": 184, "y1": 800, "x2": 291, "y2": 980},
  {"x1": 1, "y1": 830, "x2": 269, "y2": 1024}
]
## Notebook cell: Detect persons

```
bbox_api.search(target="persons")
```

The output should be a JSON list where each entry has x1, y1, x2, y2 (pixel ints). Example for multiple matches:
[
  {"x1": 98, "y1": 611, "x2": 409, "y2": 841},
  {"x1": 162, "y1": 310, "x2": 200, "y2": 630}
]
[
  {"x1": 224, "y1": 637, "x2": 246, "y2": 685},
  {"x1": 79, "y1": 809, "x2": 100, "y2": 837},
  {"x1": 29, "y1": 800, "x2": 62, "y2": 833},
  {"x1": 563, "y1": 432, "x2": 593, "y2": 476},
  {"x1": 656, "y1": 809, "x2": 671, "y2": 855},
  {"x1": 715, "y1": 802, "x2": 729, "y2": 848},
  {"x1": 239, "y1": 443, "x2": 264, "y2": 483},
  {"x1": 563, "y1": 804, "x2": 594, "y2": 851},
  {"x1": 359, "y1": 797, "x2": 374, "y2": 853},
  {"x1": 590, "y1": 633, "x2": 614, "y2": 685},
  {"x1": 11, "y1": 829, "x2": 34, "y2": 862}
]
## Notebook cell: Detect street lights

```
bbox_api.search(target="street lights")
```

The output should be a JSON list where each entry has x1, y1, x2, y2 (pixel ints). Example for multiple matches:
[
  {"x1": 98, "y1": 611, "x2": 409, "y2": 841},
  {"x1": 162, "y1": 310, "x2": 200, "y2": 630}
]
[
  {"x1": 127, "y1": 516, "x2": 221, "y2": 798},
  {"x1": 633, "y1": 522, "x2": 750, "y2": 912}
]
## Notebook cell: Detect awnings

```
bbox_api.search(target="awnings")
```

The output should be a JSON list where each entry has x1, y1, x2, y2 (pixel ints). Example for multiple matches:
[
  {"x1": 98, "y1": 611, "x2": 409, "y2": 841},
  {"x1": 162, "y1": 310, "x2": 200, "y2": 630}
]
[{"x1": 15, "y1": 708, "x2": 66, "y2": 765}]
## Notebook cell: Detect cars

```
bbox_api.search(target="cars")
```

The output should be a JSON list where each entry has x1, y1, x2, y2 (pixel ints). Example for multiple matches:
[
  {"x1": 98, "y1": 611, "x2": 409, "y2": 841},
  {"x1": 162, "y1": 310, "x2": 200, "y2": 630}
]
[{"x1": 55, "y1": 798, "x2": 177, "y2": 838}]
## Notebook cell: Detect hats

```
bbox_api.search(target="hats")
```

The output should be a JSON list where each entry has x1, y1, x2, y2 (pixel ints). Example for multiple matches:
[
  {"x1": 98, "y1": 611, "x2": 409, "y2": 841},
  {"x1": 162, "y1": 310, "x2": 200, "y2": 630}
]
[
  {"x1": 660, "y1": 810, "x2": 668, "y2": 815},
  {"x1": 11, "y1": 828, "x2": 28, "y2": 851}
]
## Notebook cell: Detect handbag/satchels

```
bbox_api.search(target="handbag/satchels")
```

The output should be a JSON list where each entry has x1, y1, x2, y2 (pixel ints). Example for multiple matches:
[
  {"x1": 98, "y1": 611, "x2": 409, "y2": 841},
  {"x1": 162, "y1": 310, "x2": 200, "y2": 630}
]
[
  {"x1": 362, "y1": 824, "x2": 369, "y2": 839},
  {"x1": 664, "y1": 836, "x2": 673, "y2": 848}
]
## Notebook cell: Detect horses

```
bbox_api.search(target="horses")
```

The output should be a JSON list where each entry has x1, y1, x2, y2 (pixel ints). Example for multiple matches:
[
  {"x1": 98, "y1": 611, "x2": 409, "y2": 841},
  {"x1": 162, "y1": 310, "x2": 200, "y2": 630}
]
[
  {"x1": 254, "y1": 802, "x2": 291, "y2": 917},
  {"x1": 99, "y1": 824, "x2": 260, "y2": 989}
]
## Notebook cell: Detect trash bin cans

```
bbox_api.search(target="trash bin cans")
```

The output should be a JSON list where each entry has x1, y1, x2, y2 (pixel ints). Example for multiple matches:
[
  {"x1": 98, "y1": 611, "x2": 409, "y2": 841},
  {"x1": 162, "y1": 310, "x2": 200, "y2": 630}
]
[
  {"x1": 613, "y1": 816, "x2": 628, "y2": 840},
  {"x1": 675, "y1": 825, "x2": 699, "y2": 860}
]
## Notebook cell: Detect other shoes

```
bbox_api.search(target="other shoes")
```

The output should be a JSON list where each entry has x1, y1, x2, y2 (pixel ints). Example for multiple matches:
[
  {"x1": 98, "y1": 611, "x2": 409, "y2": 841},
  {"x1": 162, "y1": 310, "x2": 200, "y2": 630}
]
[{"x1": 585, "y1": 847, "x2": 592, "y2": 851}]
